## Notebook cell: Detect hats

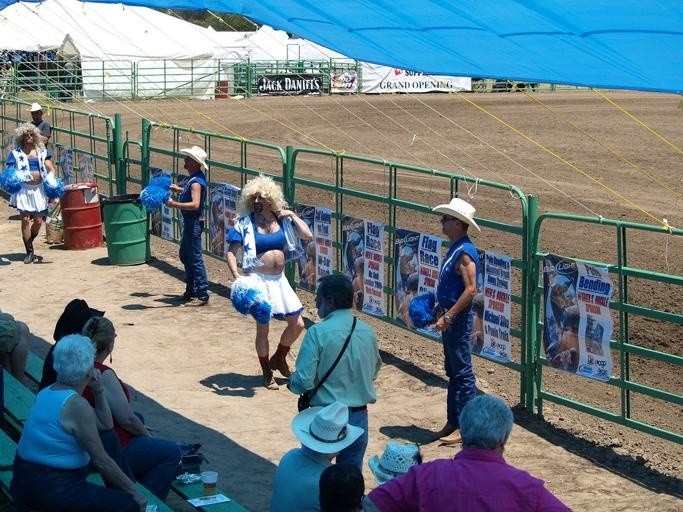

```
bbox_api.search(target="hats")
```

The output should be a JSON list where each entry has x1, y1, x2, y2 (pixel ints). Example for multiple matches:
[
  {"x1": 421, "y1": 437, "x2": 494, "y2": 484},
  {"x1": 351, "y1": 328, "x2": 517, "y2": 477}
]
[
  {"x1": 292, "y1": 402, "x2": 365, "y2": 452},
  {"x1": 433, "y1": 198, "x2": 481, "y2": 233},
  {"x1": 370, "y1": 444, "x2": 420, "y2": 481},
  {"x1": 181, "y1": 146, "x2": 207, "y2": 168},
  {"x1": 27, "y1": 102, "x2": 43, "y2": 112},
  {"x1": 55, "y1": 298, "x2": 106, "y2": 340}
]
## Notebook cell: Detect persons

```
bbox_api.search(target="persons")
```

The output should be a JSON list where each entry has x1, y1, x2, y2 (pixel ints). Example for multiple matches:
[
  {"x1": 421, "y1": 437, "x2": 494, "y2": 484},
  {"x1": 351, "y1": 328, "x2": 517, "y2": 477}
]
[
  {"x1": 81, "y1": 314, "x2": 180, "y2": 504},
  {"x1": 38, "y1": 298, "x2": 107, "y2": 394},
  {"x1": 270, "y1": 401, "x2": 365, "y2": 512},
  {"x1": 318, "y1": 465, "x2": 365, "y2": 512},
  {"x1": 0, "y1": 302, "x2": 34, "y2": 388},
  {"x1": 10, "y1": 334, "x2": 148, "y2": 511},
  {"x1": 139, "y1": 146, "x2": 211, "y2": 307},
  {"x1": 0, "y1": 123, "x2": 64, "y2": 264},
  {"x1": 367, "y1": 441, "x2": 422, "y2": 487},
  {"x1": 408, "y1": 198, "x2": 483, "y2": 446},
  {"x1": 226, "y1": 174, "x2": 312, "y2": 391},
  {"x1": 288, "y1": 276, "x2": 384, "y2": 474},
  {"x1": 26, "y1": 101, "x2": 52, "y2": 149},
  {"x1": 364, "y1": 394, "x2": 576, "y2": 512}
]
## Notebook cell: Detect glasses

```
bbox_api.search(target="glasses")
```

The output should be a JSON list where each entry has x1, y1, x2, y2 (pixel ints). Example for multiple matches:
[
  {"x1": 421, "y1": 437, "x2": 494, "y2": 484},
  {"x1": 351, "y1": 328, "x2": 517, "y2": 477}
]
[
  {"x1": 442, "y1": 216, "x2": 458, "y2": 222},
  {"x1": 24, "y1": 133, "x2": 34, "y2": 137}
]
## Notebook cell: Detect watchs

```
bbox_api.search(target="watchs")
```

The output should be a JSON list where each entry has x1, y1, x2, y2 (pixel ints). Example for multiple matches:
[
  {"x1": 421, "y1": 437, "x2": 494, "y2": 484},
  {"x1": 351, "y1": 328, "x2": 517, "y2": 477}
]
[{"x1": 442, "y1": 313, "x2": 458, "y2": 327}]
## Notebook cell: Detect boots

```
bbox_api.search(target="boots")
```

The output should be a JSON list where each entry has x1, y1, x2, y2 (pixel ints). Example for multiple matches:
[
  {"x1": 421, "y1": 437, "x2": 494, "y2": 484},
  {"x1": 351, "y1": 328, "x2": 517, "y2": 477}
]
[
  {"x1": 262, "y1": 360, "x2": 279, "y2": 390},
  {"x1": 270, "y1": 347, "x2": 292, "y2": 378},
  {"x1": 24, "y1": 238, "x2": 35, "y2": 262}
]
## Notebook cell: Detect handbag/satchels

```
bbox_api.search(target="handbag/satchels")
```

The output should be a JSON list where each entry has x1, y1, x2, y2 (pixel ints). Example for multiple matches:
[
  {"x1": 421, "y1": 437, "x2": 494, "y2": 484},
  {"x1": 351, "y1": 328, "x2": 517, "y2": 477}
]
[{"x1": 298, "y1": 317, "x2": 356, "y2": 410}]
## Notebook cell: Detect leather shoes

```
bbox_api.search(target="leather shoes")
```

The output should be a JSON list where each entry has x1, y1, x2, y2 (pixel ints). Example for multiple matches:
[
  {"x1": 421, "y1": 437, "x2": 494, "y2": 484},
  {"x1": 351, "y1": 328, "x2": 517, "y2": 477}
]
[
  {"x1": 186, "y1": 298, "x2": 209, "y2": 307},
  {"x1": 441, "y1": 428, "x2": 463, "y2": 443},
  {"x1": 432, "y1": 424, "x2": 455, "y2": 440}
]
